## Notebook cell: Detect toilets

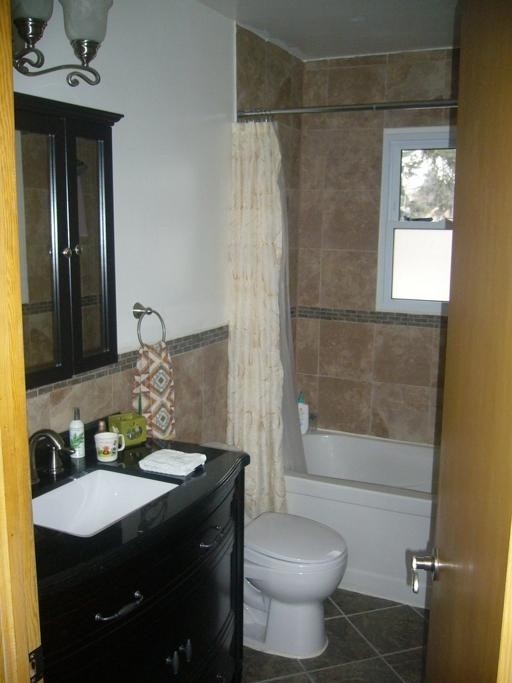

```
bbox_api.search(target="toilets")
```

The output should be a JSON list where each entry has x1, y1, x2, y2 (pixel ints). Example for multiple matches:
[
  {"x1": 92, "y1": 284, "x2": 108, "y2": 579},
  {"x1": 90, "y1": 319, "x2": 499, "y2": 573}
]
[{"x1": 244, "y1": 512, "x2": 348, "y2": 658}]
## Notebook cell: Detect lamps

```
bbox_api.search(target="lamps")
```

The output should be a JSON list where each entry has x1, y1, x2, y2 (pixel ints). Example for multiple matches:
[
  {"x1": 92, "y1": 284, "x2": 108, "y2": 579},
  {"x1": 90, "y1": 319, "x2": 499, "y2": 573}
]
[{"x1": 9, "y1": 0, "x2": 116, "y2": 91}]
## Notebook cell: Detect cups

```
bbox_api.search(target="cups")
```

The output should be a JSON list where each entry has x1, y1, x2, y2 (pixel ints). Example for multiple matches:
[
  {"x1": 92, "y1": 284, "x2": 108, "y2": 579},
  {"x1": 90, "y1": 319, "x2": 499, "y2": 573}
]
[{"x1": 94, "y1": 431, "x2": 126, "y2": 463}]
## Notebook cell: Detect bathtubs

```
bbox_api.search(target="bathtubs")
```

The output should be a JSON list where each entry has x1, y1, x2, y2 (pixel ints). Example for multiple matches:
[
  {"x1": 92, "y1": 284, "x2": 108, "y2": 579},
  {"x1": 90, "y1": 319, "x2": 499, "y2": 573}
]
[{"x1": 282, "y1": 428, "x2": 440, "y2": 609}]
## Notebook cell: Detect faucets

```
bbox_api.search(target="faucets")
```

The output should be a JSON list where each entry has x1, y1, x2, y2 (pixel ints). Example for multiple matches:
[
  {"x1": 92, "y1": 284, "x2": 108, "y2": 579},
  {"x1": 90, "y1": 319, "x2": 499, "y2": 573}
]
[{"x1": 29, "y1": 429, "x2": 75, "y2": 485}]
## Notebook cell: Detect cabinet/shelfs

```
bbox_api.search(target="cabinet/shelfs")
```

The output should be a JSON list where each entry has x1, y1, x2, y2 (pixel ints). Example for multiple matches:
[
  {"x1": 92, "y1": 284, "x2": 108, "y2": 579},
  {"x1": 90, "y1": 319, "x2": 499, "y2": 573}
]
[
  {"x1": 24, "y1": 410, "x2": 253, "y2": 682},
  {"x1": 12, "y1": 92, "x2": 127, "y2": 394}
]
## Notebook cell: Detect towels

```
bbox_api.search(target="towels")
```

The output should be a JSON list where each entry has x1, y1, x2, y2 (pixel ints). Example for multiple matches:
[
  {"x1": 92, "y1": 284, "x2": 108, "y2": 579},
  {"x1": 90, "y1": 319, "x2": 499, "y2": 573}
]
[{"x1": 128, "y1": 340, "x2": 180, "y2": 442}]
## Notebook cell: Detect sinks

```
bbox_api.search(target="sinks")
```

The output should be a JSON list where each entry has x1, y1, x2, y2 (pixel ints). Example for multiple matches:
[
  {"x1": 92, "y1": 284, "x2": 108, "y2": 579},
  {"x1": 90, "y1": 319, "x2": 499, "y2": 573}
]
[{"x1": 33, "y1": 469, "x2": 179, "y2": 537}]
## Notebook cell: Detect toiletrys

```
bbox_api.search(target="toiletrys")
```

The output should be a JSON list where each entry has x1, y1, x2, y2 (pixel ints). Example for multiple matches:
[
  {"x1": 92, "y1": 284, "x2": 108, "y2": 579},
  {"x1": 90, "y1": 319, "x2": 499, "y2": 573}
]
[{"x1": 297, "y1": 392, "x2": 309, "y2": 435}]
